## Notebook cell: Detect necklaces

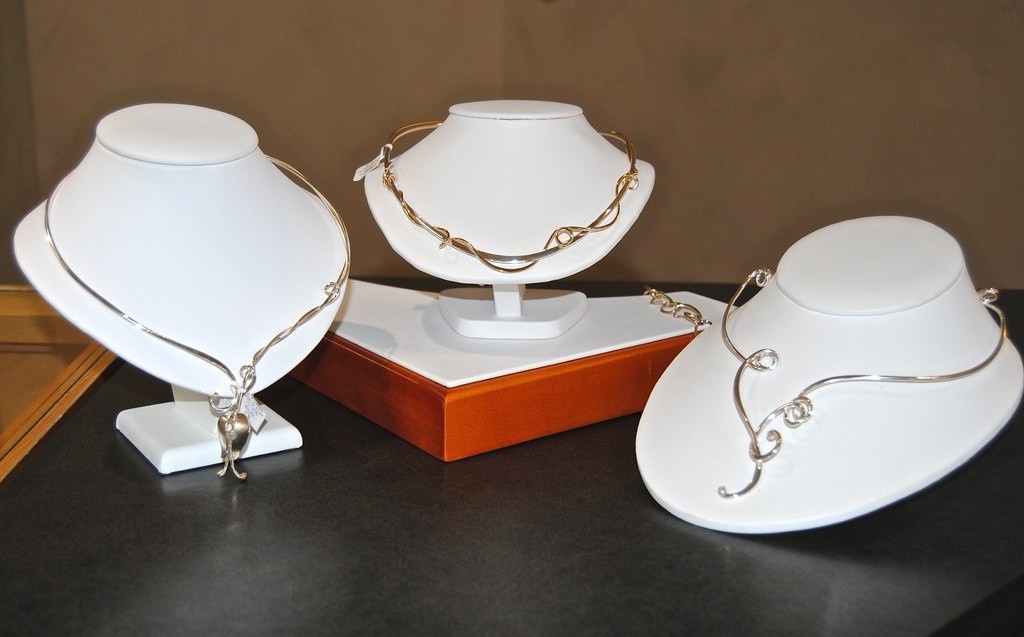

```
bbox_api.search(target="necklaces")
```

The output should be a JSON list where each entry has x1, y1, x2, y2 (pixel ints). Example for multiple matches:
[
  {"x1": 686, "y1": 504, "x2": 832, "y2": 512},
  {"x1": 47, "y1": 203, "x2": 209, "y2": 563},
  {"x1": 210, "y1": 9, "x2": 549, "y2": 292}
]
[
  {"x1": 382, "y1": 122, "x2": 639, "y2": 274},
  {"x1": 45, "y1": 153, "x2": 352, "y2": 479},
  {"x1": 717, "y1": 269, "x2": 1007, "y2": 500}
]
